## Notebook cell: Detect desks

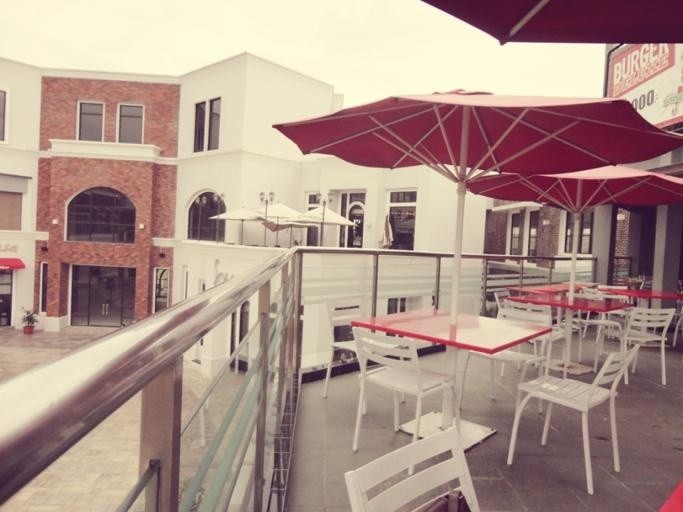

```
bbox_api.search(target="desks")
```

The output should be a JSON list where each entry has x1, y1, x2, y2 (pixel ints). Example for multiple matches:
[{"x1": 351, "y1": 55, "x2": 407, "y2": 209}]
[{"x1": 351, "y1": 310, "x2": 553, "y2": 453}]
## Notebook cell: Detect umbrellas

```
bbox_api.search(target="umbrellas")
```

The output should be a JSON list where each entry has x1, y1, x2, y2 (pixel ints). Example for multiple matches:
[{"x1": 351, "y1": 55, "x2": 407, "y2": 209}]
[
  {"x1": 270, "y1": 87, "x2": 683, "y2": 326},
  {"x1": 208, "y1": 200, "x2": 359, "y2": 247},
  {"x1": 422, "y1": 0, "x2": 683, "y2": 46},
  {"x1": 463, "y1": 165, "x2": 683, "y2": 304}
]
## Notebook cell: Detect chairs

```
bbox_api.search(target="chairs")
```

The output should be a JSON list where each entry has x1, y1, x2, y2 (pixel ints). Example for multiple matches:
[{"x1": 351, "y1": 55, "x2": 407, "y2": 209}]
[
  {"x1": 458, "y1": 309, "x2": 552, "y2": 417},
  {"x1": 352, "y1": 326, "x2": 462, "y2": 475},
  {"x1": 506, "y1": 344, "x2": 640, "y2": 495},
  {"x1": 323, "y1": 294, "x2": 406, "y2": 414},
  {"x1": 344, "y1": 425, "x2": 482, "y2": 512},
  {"x1": 494, "y1": 281, "x2": 682, "y2": 386}
]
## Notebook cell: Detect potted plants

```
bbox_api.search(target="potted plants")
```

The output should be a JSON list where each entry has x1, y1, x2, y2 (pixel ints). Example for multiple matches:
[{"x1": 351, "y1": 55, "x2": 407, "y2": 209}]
[{"x1": 20, "y1": 310, "x2": 39, "y2": 334}]
